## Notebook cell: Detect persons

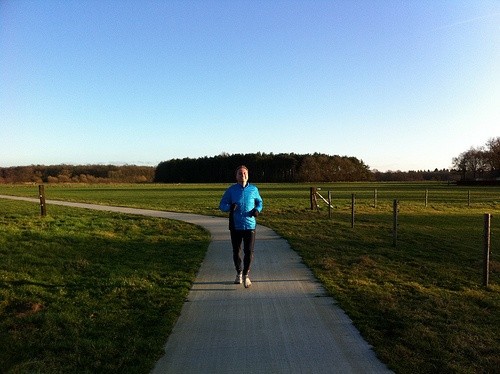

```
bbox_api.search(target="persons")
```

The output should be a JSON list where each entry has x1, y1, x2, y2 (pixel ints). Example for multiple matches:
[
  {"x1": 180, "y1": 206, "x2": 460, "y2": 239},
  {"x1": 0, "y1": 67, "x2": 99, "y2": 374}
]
[{"x1": 218, "y1": 165, "x2": 263, "y2": 288}]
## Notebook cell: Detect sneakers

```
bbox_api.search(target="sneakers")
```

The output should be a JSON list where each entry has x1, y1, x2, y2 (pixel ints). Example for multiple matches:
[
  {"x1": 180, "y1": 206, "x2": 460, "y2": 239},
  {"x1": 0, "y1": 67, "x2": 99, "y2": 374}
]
[
  {"x1": 243, "y1": 275, "x2": 251, "y2": 288},
  {"x1": 235, "y1": 274, "x2": 242, "y2": 284}
]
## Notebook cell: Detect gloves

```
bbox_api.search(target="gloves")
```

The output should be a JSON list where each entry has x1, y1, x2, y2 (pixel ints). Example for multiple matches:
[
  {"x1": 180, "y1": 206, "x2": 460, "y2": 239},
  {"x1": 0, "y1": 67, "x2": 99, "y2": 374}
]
[
  {"x1": 231, "y1": 203, "x2": 236, "y2": 210},
  {"x1": 250, "y1": 210, "x2": 258, "y2": 217}
]
